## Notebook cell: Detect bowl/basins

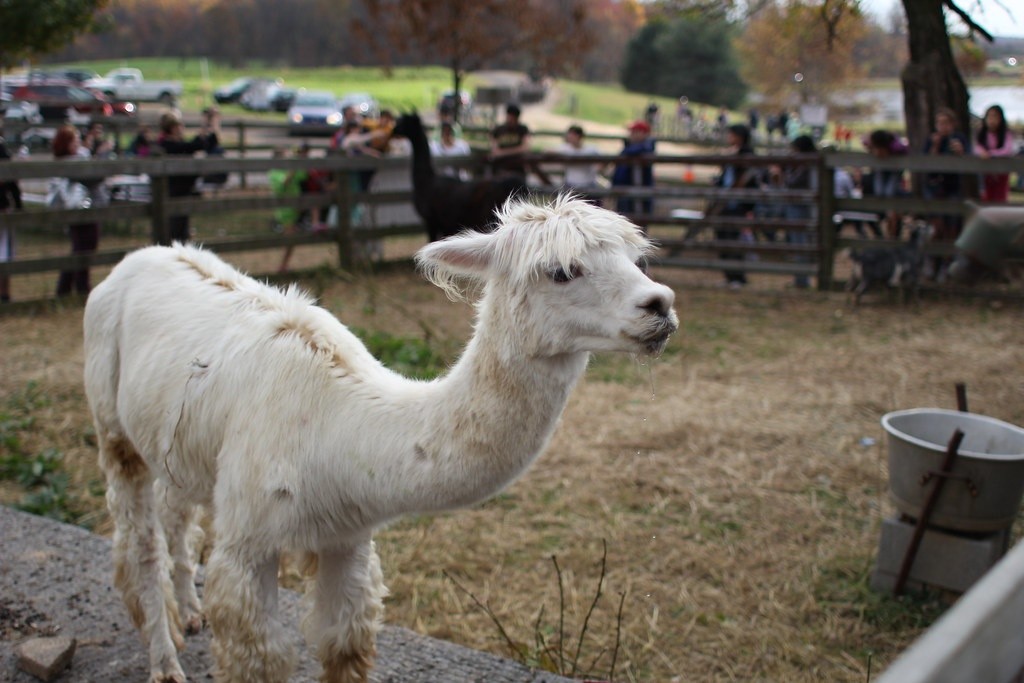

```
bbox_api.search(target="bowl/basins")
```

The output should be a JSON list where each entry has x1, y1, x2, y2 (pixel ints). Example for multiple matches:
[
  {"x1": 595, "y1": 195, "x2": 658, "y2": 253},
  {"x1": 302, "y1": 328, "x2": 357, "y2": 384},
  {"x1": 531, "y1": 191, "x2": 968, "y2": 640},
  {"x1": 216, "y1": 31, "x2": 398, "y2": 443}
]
[{"x1": 881, "y1": 408, "x2": 1024, "y2": 530}]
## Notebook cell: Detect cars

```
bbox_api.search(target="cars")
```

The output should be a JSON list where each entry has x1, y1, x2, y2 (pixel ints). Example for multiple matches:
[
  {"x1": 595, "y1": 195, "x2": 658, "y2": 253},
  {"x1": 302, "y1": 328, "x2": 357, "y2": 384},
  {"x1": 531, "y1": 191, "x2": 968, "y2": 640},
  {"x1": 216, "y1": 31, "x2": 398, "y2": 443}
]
[
  {"x1": 287, "y1": 90, "x2": 344, "y2": 139},
  {"x1": 1, "y1": 67, "x2": 134, "y2": 118},
  {"x1": 219, "y1": 74, "x2": 292, "y2": 113}
]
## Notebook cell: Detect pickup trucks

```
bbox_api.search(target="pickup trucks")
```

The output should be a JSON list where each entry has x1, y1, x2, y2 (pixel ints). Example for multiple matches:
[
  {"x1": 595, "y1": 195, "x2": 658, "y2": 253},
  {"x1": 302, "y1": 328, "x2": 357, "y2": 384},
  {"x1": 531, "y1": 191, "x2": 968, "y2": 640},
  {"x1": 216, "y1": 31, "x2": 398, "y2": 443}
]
[{"x1": 81, "y1": 67, "x2": 185, "y2": 107}]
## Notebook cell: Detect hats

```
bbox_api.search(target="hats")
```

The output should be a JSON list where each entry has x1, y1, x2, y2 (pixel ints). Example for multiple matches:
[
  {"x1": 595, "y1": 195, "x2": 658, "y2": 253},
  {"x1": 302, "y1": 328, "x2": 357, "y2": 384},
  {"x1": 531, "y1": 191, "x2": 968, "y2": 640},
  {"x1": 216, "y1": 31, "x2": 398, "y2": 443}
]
[{"x1": 628, "y1": 119, "x2": 650, "y2": 134}]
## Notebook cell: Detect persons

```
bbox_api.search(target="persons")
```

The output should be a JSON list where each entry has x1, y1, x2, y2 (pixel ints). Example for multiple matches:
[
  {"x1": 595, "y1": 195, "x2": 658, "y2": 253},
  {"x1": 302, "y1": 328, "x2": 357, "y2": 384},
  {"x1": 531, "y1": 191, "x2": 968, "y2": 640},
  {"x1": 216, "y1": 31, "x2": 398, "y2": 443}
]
[
  {"x1": 611, "y1": 115, "x2": 655, "y2": 237},
  {"x1": 193, "y1": 105, "x2": 230, "y2": 190},
  {"x1": 269, "y1": 100, "x2": 359, "y2": 237},
  {"x1": 330, "y1": 105, "x2": 367, "y2": 155},
  {"x1": 645, "y1": 89, "x2": 1016, "y2": 297},
  {"x1": 40, "y1": 86, "x2": 183, "y2": 195},
  {"x1": 486, "y1": 101, "x2": 531, "y2": 203},
  {"x1": 544, "y1": 123, "x2": 610, "y2": 196},
  {"x1": 51, "y1": 127, "x2": 114, "y2": 304},
  {"x1": 361, "y1": 111, "x2": 396, "y2": 151},
  {"x1": 150, "y1": 112, "x2": 218, "y2": 246},
  {"x1": 0, "y1": 107, "x2": 23, "y2": 306},
  {"x1": 315, "y1": 120, "x2": 385, "y2": 235},
  {"x1": 429, "y1": 121, "x2": 473, "y2": 179}
]
